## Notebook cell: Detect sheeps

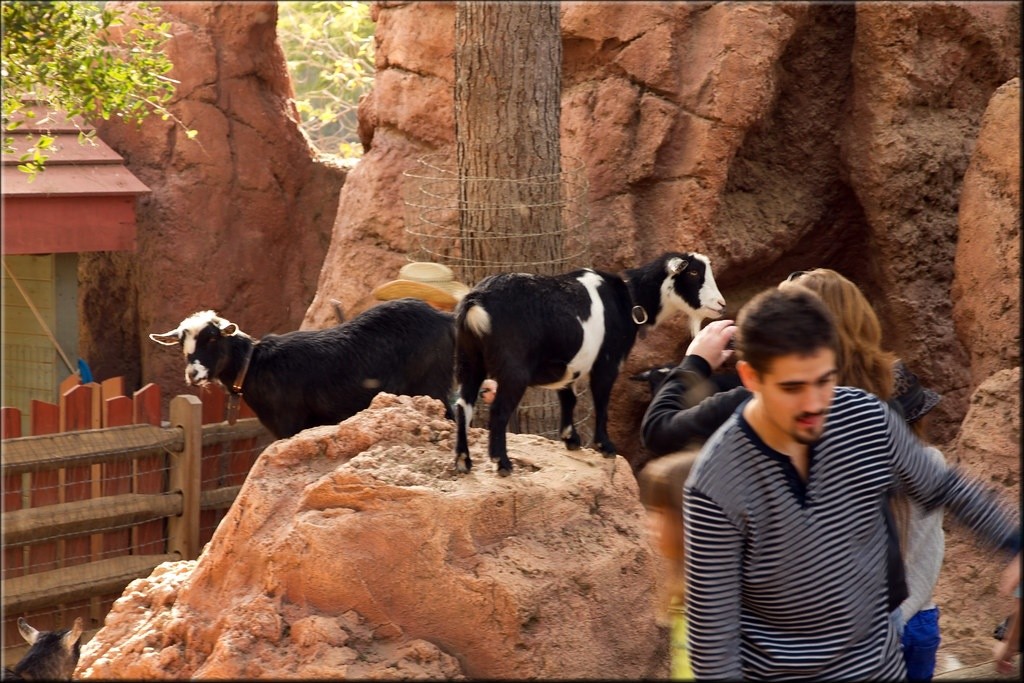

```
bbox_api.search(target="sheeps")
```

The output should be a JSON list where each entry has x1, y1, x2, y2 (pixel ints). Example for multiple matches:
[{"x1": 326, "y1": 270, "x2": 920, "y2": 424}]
[
  {"x1": 149, "y1": 249, "x2": 726, "y2": 477},
  {"x1": 0, "y1": 613, "x2": 100, "y2": 683}
]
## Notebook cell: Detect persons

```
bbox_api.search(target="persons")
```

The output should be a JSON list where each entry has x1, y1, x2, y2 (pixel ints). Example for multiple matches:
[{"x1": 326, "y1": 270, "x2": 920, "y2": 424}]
[
  {"x1": 642, "y1": 268, "x2": 910, "y2": 635},
  {"x1": 683, "y1": 287, "x2": 1024, "y2": 683}
]
